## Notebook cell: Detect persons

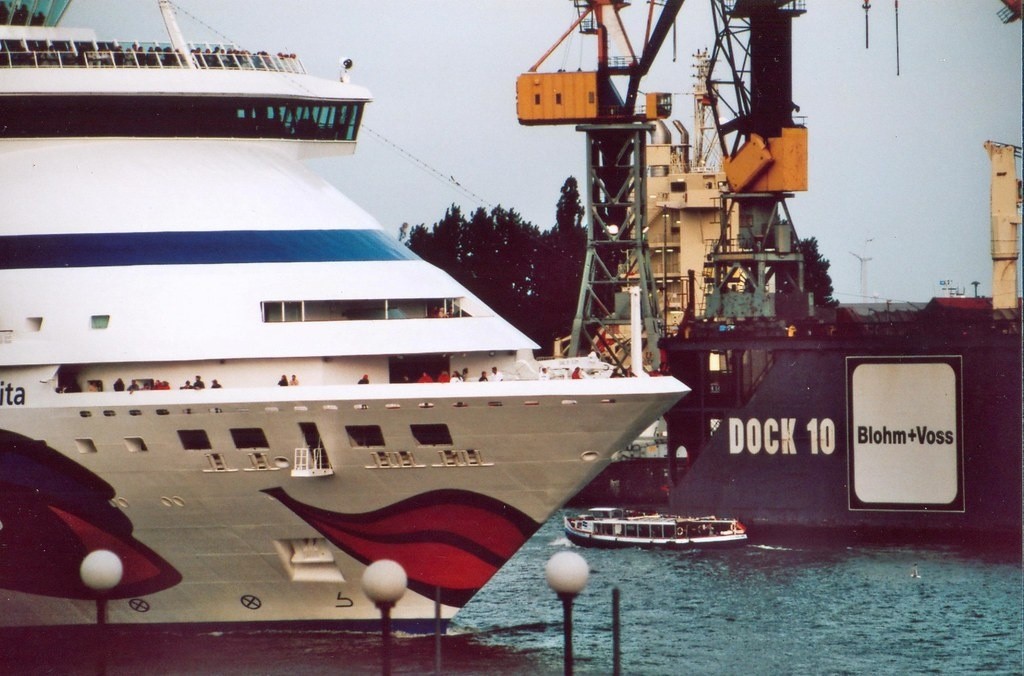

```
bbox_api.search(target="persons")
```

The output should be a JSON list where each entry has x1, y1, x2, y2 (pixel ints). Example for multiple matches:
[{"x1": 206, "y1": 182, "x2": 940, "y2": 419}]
[
  {"x1": 0, "y1": 44, "x2": 298, "y2": 71},
  {"x1": 610, "y1": 367, "x2": 639, "y2": 378},
  {"x1": 357, "y1": 374, "x2": 369, "y2": 384},
  {"x1": 0, "y1": 0, "x2": 46, "y2": 26},
  {"x1": 277, "y1": 374, "x2": 299, "y2": 386},
  {"x1": 571, "y1": 366, "x2": 582, "y2": 379},
  {"x1": 538, "y1": 366, "x2": 550, "y2": 380},
  {"x1": 398, "y1": 366, "x2": 503, "y2": 383},
  {"x1": 56, "y1": 374, "x2": 221, "y2": 395},
  {"x1": 439, "y1": 308, "x2": 449, "y2": 318}
]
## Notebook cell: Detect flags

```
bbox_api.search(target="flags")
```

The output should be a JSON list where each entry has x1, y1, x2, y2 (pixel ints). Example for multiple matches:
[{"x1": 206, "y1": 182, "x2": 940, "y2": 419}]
[{"x1": 736, "y1": 521, "x2": 748, "y2": 531}]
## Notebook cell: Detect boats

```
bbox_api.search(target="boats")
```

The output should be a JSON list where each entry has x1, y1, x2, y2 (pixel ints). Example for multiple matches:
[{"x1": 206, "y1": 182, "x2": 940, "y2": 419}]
[{"x1": 560, "y1": 506, "x2": 748, "y2": 553}]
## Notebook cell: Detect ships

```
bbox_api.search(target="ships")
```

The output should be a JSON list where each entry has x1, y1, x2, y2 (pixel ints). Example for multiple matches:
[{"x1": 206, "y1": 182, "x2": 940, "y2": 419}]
[
  {"x1": 514, "y1": 2, "x2": 1023, "y2": 554},
  {"x1": 0, "y1": 1, "x2": 694, "y2": 639}
]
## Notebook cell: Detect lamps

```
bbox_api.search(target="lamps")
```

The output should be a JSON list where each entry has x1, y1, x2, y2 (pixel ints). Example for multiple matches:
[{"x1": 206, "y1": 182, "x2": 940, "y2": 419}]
[
  {"x1": 545, "y1": 550, "x2": 590, "y2": 596},
  {"x1": 359, "y1": 561, "x2": 410, "y2": 602},
  {"x1": 80, "y1": 550, "x2": 123, "y2": 590}
]
